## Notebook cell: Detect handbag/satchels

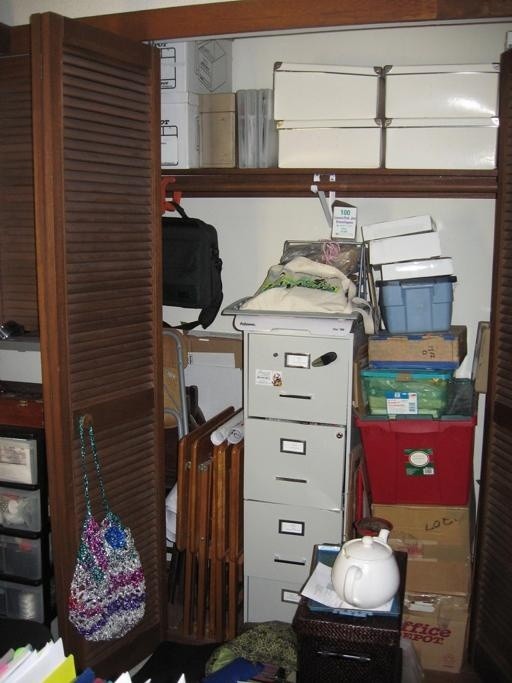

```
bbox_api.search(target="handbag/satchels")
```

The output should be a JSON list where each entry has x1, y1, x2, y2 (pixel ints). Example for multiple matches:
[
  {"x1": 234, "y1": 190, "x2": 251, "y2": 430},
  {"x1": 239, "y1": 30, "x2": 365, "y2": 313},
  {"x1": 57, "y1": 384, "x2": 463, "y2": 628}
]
[{"x1": 70, "y1": 513, "x2": 146, "y2": 641}]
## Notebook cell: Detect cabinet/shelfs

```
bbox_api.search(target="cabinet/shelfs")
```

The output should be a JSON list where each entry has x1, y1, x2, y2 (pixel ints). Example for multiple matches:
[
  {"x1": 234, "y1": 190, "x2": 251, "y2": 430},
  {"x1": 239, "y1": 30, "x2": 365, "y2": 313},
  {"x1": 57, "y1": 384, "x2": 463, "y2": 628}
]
[
  {"x1": 242, "y1": 331, "x2": 367, "y2": 631},
  {"x1": 0, "y1": 11, "x2": 168, "y2": 683}
]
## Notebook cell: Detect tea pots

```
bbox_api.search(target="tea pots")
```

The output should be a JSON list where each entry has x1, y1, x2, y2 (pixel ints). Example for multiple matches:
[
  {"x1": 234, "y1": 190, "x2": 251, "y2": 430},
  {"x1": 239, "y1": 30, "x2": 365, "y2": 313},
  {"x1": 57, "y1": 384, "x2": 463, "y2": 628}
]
[{"x1": 331, "y1": 529, "x2": 400, "y2": 609}]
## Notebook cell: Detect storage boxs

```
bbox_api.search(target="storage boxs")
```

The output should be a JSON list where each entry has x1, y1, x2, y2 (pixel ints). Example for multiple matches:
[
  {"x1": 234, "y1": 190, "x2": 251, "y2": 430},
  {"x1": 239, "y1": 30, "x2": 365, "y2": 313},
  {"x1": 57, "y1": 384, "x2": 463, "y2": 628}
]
[
  {"x1": 276, "y1": 119, "x2": 382, "y2": 169},
  {"x1": 367, "y1": 502, "x2": 477, "y2": 600},
  {"x1": 365, "y1": 324, "x2": 469, "y2": 367},
  {"x1": 0, "y1": 331, "x2": 56, "y2": 625},
  {"x1": 384, "y1": 115, "x2": 498, "y2": 170},
  {"x1": 271, "y1": 60, "x2": 383, "y2": 119},
  {"x1": 356, "y1": 366, "x2": 453, "y2": 416},
  {"x1": 382, "y1": 62, "x2": 503, "y2": 119},
  {"x1": 375, "y1": 276, "x2": 457, "y2": 334},
  {"x1": 356, "y1": 378, "x2": 477, "y2": 509},
  {"x1": 401, "y1": 593, "x2": 471, "y2": 675}
]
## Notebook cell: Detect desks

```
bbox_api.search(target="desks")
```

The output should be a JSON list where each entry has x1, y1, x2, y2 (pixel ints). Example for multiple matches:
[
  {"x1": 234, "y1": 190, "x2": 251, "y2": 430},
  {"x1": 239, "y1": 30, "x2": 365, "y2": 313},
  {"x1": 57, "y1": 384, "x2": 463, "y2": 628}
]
[{"x1": 176, "y1": 406, "x2": 243, "y2": 642}]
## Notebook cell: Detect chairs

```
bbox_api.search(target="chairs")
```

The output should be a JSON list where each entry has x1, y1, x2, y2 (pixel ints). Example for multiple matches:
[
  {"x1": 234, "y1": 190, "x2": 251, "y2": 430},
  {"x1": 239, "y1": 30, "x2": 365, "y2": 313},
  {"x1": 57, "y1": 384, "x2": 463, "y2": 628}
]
[{"x1": 161, "y1": 325, "x2": 189, "y2": 439}]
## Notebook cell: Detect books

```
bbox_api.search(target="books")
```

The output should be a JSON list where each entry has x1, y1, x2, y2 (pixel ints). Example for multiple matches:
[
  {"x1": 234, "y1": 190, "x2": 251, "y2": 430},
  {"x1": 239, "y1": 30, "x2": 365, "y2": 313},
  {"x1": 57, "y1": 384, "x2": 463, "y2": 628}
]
[
  {"x1": 236, "y1": 88, "x2": 278, "y2": 168},
  {"x1": 0, "y1": 637, "x2": 186, "y2": 683}
]
